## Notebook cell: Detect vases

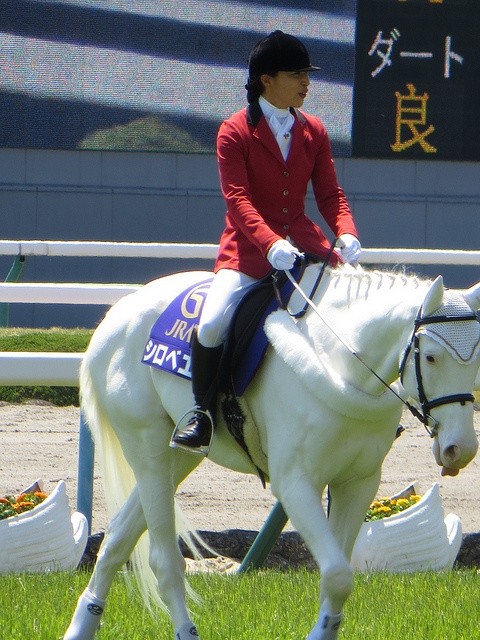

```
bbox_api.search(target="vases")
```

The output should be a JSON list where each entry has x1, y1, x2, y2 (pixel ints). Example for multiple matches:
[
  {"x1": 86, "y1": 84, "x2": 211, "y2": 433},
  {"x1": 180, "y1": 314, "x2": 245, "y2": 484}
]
[
  {"x1": 0, "y1": 481, "x2": 88, "y2": 576},
  {"x1": 349, "y1": 481, "x2": 464, "y2": 574}
]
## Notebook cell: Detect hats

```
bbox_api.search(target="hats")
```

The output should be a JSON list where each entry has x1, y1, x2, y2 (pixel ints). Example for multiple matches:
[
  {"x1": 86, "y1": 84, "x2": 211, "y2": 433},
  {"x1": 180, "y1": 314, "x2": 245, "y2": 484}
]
[{"x1": 249, "y1": 29, "x2": 321, "y2": 72}]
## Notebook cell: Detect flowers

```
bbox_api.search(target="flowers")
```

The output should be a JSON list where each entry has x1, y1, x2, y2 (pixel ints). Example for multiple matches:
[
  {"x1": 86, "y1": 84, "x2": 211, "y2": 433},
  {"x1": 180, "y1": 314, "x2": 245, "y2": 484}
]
[
  {"x1": 0, "y1": 489, "x2": 49, "y2": 520},
  {"x1": 362, "y1": 494, "x2": 426, "y2": 523}
]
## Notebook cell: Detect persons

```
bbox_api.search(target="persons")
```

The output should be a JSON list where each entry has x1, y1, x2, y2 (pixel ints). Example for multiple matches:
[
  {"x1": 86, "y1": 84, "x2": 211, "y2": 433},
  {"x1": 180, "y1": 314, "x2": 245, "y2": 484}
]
[{"x1": 173, "y1": 30, "x2": 363, "y2": 447}]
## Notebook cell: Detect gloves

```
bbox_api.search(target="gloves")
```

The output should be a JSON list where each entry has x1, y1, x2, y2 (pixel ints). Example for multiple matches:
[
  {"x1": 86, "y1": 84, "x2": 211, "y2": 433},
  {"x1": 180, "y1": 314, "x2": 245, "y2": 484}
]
[
  {"x1": 266, "y1": 238, "x2": 304, "y2": 271},
  {"x1": 335, "y1": 235, "x2": 362, "y2": 263}
]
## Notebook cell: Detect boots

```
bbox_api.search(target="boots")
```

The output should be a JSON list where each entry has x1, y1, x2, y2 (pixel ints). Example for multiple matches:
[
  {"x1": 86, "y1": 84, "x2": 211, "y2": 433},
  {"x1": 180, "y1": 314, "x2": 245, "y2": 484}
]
[{"x1": 172, "y1": 328, "x2": 224, "y2": 448}]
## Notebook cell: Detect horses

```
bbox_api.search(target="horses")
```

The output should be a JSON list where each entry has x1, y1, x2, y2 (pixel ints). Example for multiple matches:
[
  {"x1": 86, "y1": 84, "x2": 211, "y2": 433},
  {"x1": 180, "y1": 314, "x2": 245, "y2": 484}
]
[{"x1": 65, "y1": 266, "x2": 480, "y2": 640}]
